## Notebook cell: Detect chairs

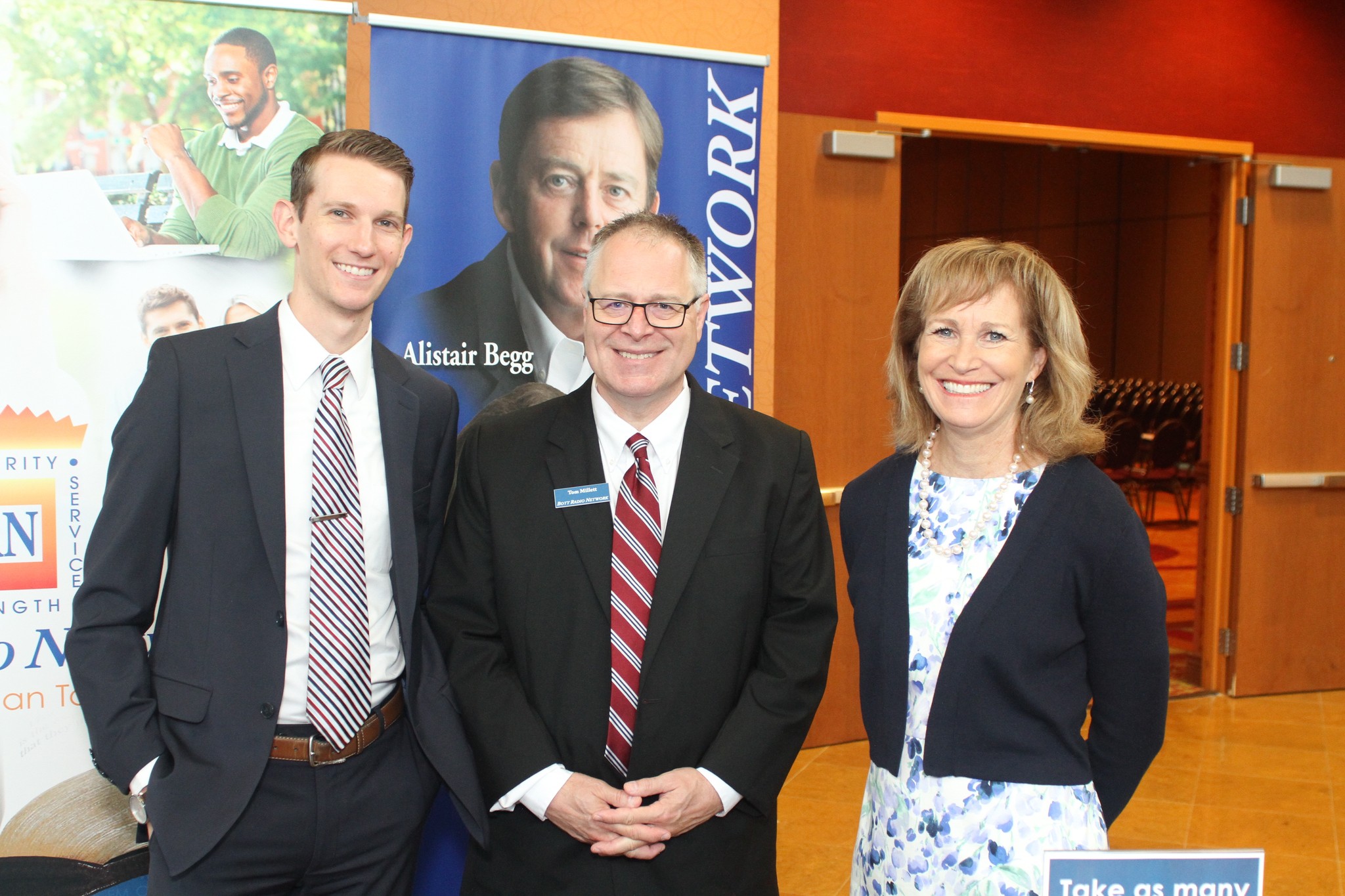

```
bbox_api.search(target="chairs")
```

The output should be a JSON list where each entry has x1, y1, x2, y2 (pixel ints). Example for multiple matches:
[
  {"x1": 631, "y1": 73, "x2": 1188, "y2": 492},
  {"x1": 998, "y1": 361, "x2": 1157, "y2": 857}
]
[{"x1": 1080, "y1": 374, "x2": 1203, "y2": 532}]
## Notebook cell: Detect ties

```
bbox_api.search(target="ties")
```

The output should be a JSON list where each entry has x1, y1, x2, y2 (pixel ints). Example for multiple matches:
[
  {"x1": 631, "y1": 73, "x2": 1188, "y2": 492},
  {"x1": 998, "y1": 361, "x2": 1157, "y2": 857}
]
[
  {"x1": 603, "y1": 432, "x2": 662, "y2": 788},
  {"x1": 303, "y1": 357, "x2": 373, "y2": 754}
]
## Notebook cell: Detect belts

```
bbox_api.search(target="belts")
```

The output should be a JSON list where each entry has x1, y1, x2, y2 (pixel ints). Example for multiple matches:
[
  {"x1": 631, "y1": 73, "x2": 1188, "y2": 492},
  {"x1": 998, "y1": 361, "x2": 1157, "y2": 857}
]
[{"x1": 267, "y1": 683, "x2": 406, "y2": 767}]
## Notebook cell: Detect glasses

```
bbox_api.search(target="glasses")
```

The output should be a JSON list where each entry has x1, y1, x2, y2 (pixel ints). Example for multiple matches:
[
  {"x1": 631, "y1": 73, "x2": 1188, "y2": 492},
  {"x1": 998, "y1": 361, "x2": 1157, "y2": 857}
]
[{"x1": 587, "y1": 289, "x2": 703, "y2": 329}]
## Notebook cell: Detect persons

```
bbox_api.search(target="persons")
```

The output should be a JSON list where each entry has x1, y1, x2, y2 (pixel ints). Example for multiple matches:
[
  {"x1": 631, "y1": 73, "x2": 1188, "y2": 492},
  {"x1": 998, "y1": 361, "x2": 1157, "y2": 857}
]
[
  {"x1": 120, "y1": 29, "x2": 333, "y2": 263},
  {"x1": 62, "y1": 129, "x2": 457, "y2": 896},
  {"x1": 141, "y1": 287, "x2": 208, "y2": 339},
  {"x1": 393, "y1": 57, "x2": 665, "y2": 415},
  {"x1": 834, "y1": 237, "x2": 1170, "y2": 895},
  {"x1": 433, "y1": 211, "x2": 838, "y2": 895}
]
